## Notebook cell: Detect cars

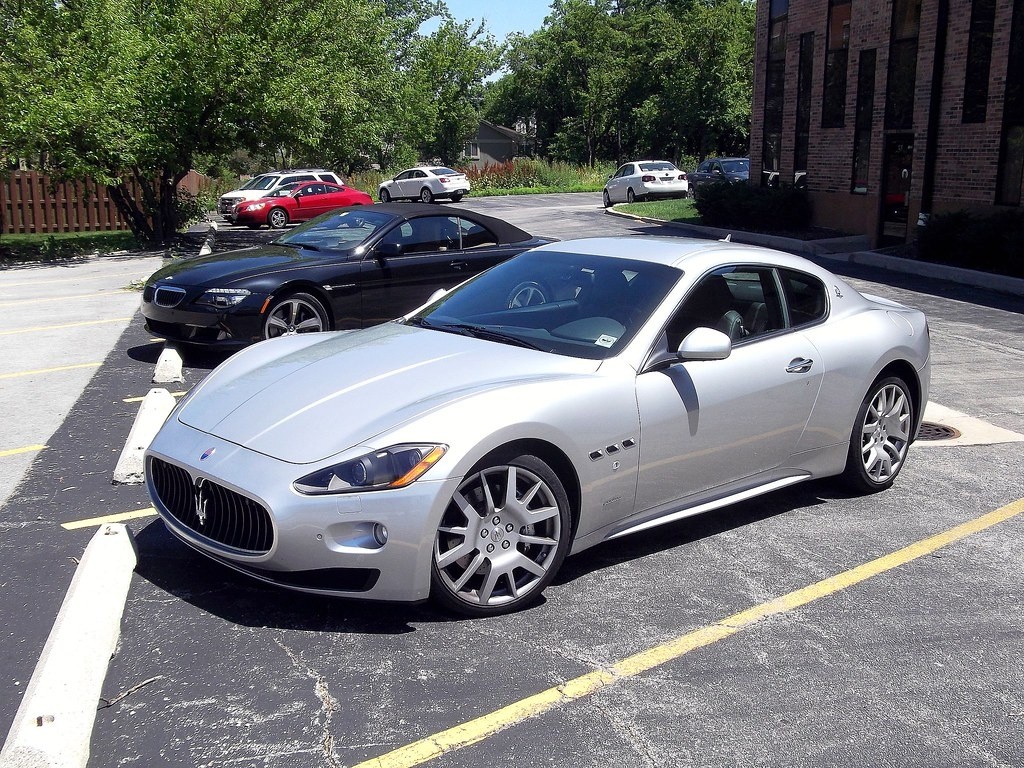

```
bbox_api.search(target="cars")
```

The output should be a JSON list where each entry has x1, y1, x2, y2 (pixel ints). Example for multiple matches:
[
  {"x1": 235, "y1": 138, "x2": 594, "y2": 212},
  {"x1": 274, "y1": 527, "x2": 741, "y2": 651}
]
[
  {"x1": 140, "y1": 236, "x2": 933, "y2": 618},
  {"x1": 763, "y1": 170, "x2": 807, "y2": 190},
  {"x1": 602, "y1": 159, "x2": 688, "y2": 207},
  {"x1": 231, "y1": 179, "x2": 375, "y2": 229},
  {"x1": 856, "y1": 180, "x2": 907, "y2": 221},
  {"x1": 139, "y1": 203, "x2": 562, "y2": 362},
  {"x1": 377, "y1": 165, "x2": 472, "y2": 204},
  {"x1": 686, "y1": 157, "x2": 749, "y2": 198}
]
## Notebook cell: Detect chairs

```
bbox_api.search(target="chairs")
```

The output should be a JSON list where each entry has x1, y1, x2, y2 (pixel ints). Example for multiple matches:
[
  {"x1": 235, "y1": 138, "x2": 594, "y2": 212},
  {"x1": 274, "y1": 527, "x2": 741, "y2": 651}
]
[
  {"x1": 373, "y1": 222, "x2": 402, "y2": 242},
  {"x1": 574, "y1": 268, "x2": 633, "y2": 324},
  {"x1": 412, "y1": 219, "x2": 448, "y2": 253},
  {"x1": 667, "y1": 274, "x2": 744, "y2": 351}
]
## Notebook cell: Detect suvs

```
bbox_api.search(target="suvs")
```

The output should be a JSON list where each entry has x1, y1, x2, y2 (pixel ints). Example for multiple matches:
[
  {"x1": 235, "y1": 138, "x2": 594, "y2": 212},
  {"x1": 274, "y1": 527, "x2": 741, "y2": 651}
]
[{"x1": 217, "y1": 167, "x2": 345, "y2": 216}]
